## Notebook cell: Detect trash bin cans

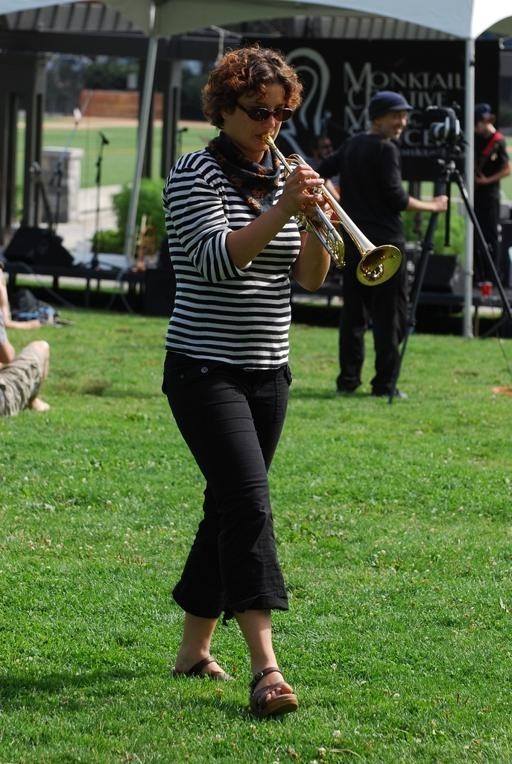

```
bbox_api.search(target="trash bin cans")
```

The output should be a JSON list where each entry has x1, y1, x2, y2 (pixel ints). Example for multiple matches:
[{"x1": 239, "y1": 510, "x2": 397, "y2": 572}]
[{"x1": 40, "y1": 146, "x2": 83, "y2": 223}]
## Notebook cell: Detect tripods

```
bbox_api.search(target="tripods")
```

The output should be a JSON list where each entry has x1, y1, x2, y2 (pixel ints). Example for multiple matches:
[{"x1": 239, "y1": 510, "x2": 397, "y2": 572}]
[
  {"x1": 32, "y1": 173, "x2": 54, "y2": 230},
  {"x1": 386, "y1": 157, "x2": 511, "y2": 404}
]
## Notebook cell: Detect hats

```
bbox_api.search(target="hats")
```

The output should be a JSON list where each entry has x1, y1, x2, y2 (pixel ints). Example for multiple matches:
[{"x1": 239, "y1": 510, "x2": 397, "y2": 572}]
[
  {"x1": 368, "y1": 90, "x2": 413, "y2": 121},
  {"x1": 475, "y1": 102, "x2": 491, "y2": 113}
]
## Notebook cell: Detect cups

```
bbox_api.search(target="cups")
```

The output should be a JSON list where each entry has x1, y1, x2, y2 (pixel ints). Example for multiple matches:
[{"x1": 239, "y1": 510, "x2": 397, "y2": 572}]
[{"x1": 480, "y1": 281, "x2": 493, "y2": 303}]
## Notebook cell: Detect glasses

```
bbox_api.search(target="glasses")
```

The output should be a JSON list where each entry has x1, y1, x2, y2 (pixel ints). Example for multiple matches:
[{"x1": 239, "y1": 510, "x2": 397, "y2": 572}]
[{"x1": 234, "y1": 99, "x2": 294, "y2": 122}]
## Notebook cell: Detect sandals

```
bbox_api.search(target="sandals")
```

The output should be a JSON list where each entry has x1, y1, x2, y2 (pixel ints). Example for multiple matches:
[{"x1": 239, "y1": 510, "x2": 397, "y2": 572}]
[
  {"x1": 170, "y1": 656, "x2": 236, "y2": 681},
  {"x1": 249, "y1": 667, "x2": 299, "y2": 718}
]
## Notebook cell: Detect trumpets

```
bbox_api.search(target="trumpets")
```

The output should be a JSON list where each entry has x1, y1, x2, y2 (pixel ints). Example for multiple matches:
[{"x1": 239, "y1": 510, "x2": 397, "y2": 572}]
[
  {"x1": 130, "y1": 211, "x2": 158, "y2": 273},
  {"x1": 262, "y1": 131, "x2": 402, "y2": 286}
]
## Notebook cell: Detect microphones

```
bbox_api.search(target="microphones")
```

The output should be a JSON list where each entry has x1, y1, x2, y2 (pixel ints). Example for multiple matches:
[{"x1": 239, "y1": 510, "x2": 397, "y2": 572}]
[{"x1": 98, "y1": 132, "x2": 109, "y2": 145}]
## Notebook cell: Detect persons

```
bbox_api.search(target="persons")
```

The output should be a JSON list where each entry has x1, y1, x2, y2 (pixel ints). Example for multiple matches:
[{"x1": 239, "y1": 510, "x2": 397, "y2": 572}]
[
  {"x1": 0, "y1": 261, "x2": 51, "y2": 416},
  {"x1": 160, "y1": 41, "x2": 342, "y2": 715},
  {"x1": 314, "y1": 91, "x2": 450, "y2": 400},
  {"x1": 474, "y1": 103, "x2": 512, "y2": 288}
]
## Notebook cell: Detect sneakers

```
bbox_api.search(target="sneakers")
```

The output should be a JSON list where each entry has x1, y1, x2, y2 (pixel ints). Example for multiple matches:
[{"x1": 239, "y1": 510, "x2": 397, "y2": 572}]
[{"x1": 337, "y1": 382, "x2": 408, "y2": 399}]
